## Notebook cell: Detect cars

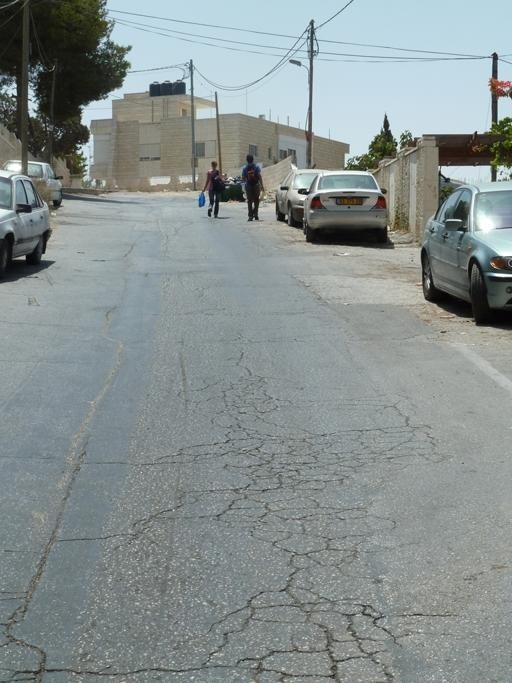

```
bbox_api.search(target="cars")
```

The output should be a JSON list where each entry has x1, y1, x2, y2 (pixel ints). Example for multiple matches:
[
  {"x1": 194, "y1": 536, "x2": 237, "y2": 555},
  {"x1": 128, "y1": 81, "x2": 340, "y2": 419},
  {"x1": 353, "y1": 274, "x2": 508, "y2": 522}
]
[
  {"x1": 276, "y1": 169, "x2": 389, "y2": 243},
  {"x1": 0, "y1": 159, "x2": 63, "y2": 279},
  {"x1": 421, "y1": 182, "x2": 512, "y2": 323}
]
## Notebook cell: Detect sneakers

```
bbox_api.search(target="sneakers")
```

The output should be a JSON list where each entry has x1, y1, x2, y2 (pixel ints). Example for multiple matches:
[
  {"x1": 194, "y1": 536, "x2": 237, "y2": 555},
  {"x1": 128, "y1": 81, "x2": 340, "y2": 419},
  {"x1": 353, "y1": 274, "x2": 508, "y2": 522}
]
[
  {"x1": 208, "y1": 208, "x2": 217, "y2": 218},
  {"x1": 248, "y1": 216, "x2": 258, "y2": 221}
]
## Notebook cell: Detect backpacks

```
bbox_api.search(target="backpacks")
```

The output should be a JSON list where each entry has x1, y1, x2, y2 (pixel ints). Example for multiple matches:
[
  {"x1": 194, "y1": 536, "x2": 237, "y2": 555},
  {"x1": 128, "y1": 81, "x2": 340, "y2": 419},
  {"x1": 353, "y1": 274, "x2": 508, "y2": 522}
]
[{"x1": 247, "y1": 164, "x2": 258, "y2": 184}]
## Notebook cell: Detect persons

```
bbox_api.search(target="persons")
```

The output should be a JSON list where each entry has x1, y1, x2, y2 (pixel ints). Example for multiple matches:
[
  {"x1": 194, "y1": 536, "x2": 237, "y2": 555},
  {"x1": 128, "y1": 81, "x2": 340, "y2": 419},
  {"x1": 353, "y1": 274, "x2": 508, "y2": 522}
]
[
  {"x1": 240, "y1": 153, "x2": 265, "y2": 220},
  {"x1": 202, "y1": 160, "x2": 222, "y2": 218}
]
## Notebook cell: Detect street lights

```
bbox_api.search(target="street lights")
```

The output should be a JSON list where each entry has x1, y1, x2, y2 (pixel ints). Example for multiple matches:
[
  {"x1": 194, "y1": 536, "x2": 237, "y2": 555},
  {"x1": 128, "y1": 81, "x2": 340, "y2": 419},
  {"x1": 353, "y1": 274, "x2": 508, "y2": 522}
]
[{"x1": 290, "y1": 59, "x2": 309, "y2": 168}]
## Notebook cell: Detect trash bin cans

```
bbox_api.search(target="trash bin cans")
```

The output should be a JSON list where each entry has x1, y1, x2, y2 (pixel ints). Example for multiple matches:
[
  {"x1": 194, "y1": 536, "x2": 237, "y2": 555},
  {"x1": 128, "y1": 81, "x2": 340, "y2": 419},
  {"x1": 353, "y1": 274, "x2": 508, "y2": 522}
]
[{"x1": 221, "y1": 180, "x2": 244, "y2": 202}]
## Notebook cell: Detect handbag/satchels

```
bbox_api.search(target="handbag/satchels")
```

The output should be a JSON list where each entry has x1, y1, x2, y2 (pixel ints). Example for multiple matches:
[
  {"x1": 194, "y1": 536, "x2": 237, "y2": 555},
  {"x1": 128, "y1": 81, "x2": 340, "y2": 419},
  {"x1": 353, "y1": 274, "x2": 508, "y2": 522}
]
[
  {"x1": 212, "y1": 175, "x2": 225, "y2": 192},
  {"x1": 199, "y1": 192, "x2": 205, "y2": 207}
]
